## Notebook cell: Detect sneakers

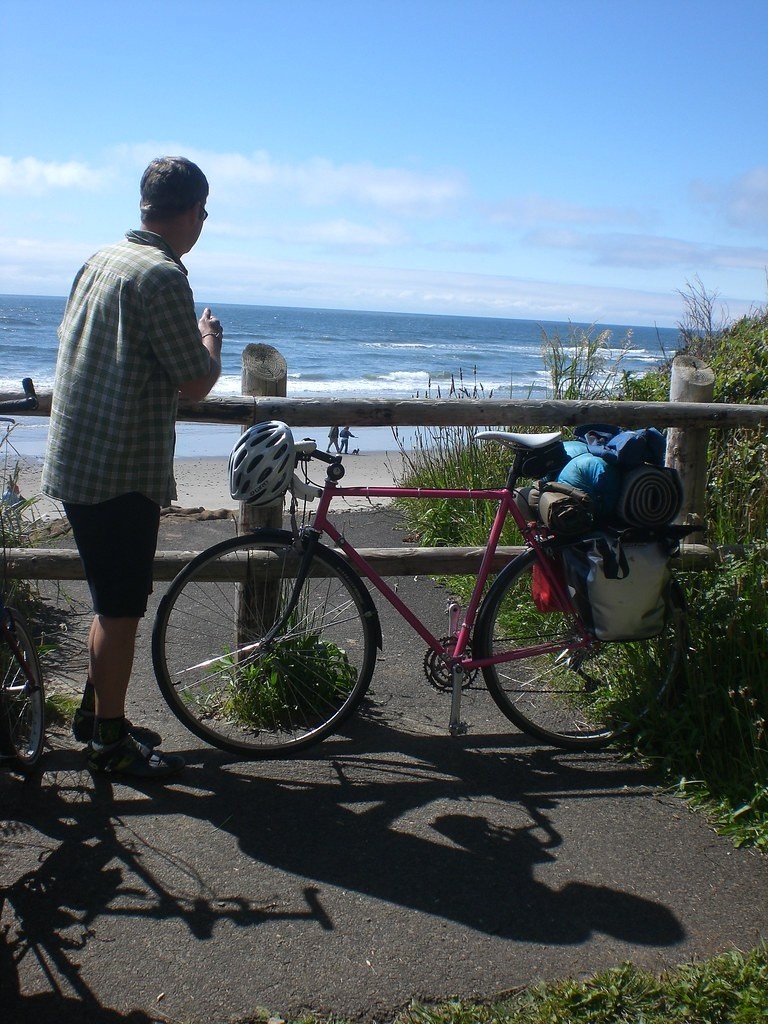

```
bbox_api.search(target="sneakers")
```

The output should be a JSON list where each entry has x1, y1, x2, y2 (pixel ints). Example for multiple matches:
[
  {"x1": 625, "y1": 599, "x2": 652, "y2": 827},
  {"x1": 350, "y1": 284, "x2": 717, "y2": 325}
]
[
  {"x1": 87, "y1": 732, "x2": 186, "y2": 778},
  {"x1": 72, "y1": 708, "x2": 162, "y2": 749}
]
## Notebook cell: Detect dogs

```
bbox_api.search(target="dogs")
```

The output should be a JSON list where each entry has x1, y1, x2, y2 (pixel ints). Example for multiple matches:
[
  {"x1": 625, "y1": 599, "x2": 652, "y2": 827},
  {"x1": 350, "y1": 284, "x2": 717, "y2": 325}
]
[{"x1": 351, "y1": 448, "x2": 360, "y2": 455}]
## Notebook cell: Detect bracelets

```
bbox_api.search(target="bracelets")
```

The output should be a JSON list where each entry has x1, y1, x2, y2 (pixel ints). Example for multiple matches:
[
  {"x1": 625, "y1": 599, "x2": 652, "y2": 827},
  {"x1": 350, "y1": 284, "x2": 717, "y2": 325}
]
[{"x1": 199, "y1": 332, "x2": 224, "y2": 341}]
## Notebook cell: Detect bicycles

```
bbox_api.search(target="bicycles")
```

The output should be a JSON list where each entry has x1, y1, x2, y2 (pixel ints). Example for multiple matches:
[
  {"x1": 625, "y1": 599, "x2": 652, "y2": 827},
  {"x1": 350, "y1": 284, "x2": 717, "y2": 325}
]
[
  {"x1": 150, "y1": 439, "x2": 706, "y2": 761},
  {"x1": 0, "y1": 376, "x2": 49, "y2": 774}
]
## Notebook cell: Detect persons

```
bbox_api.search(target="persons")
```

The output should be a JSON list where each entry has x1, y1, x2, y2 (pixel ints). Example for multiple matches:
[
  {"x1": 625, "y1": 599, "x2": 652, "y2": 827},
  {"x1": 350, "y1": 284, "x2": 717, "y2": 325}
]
[
  {"x1": 339, "y1": 425, "x2": 356, "y2": 454},
  {"x1": 42, "y1": 158, "x2": 223, "y2": 780},
  {"x1": 326, "y1": 424, "x2": 340, "y2": 453}
]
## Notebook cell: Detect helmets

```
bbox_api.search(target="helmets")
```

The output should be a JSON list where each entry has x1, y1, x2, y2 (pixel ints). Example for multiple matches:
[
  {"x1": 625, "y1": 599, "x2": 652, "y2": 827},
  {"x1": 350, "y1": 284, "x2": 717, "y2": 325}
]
[{"x1": 227, "y1": 420, "x2": 296, "y2": 508}]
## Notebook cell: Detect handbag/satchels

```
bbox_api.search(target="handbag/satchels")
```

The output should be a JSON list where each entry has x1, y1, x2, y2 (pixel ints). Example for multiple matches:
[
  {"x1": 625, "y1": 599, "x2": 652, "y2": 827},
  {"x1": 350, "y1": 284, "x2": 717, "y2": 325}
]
[
  {"x1": 573, "y1": 524, "x2": 680, "y2": 643},
  {"x1": 528, "y1": 521, "x2": 577, "y2": 612}
]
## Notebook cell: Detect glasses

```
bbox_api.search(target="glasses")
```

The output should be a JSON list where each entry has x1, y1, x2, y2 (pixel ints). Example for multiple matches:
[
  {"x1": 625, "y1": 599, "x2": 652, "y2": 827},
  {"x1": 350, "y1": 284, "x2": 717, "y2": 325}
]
[{"x1": 191, "y1": 201, "x2": 209, "y2": 221}]
